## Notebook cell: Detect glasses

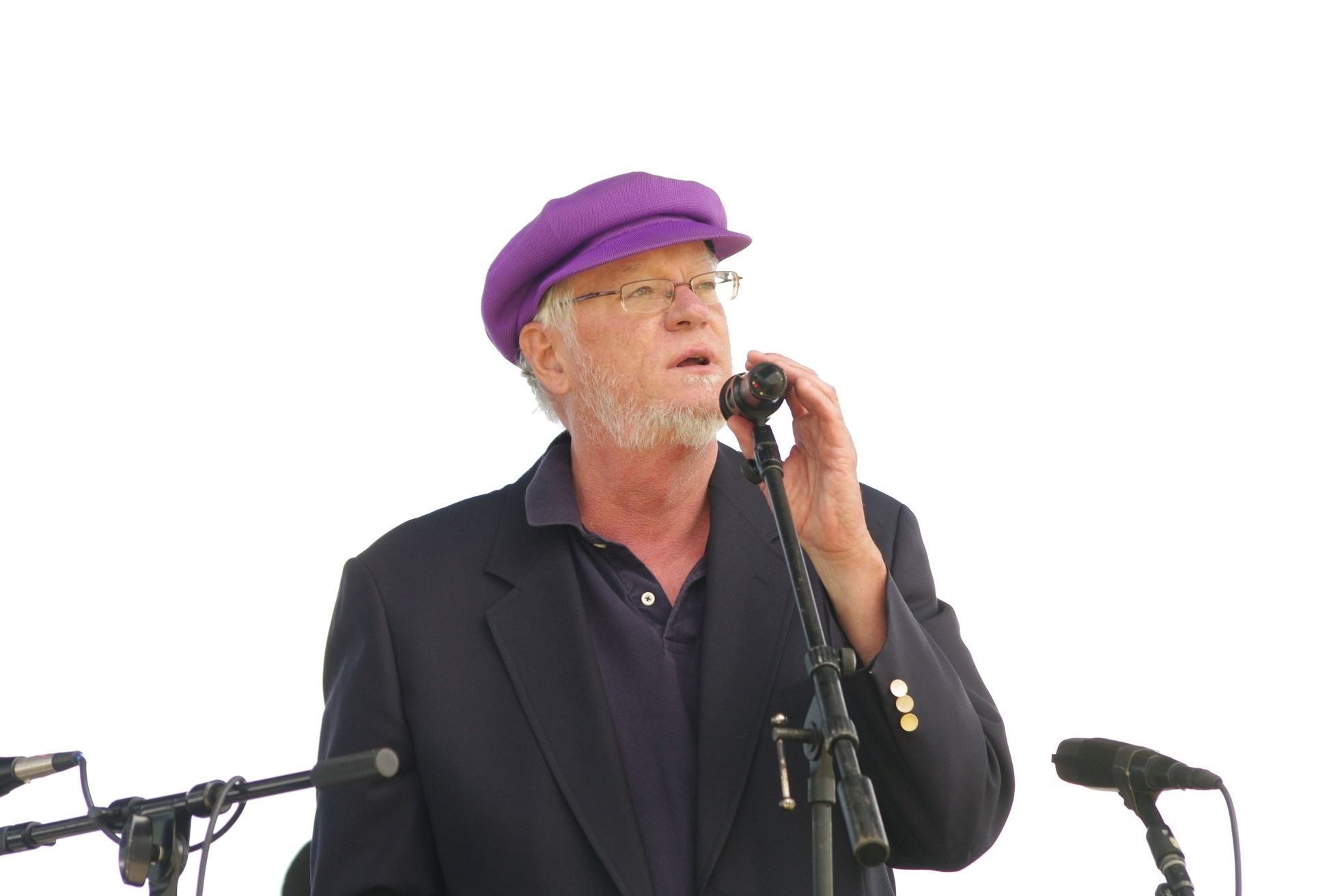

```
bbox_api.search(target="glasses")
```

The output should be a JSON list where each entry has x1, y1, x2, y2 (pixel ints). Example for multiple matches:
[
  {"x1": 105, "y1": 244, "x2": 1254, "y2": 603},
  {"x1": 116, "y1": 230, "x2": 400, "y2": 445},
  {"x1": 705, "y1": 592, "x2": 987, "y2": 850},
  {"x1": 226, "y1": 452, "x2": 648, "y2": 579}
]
[{"x1": 559, "y1": 271, "x2": 743, "y2": 313}]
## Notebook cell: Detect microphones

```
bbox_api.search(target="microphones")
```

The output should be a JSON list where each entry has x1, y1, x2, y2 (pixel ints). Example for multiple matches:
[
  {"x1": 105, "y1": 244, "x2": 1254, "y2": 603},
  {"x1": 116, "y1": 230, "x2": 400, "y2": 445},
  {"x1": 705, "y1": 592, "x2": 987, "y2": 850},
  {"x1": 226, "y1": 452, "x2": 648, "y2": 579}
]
[
  {"x1": 0, "y1": 751, "x2": 83, "y2": 798},
  {"x1": 1052, "y1": 738, "x2": 1222, "y2": 791},
  {"x1": 719, "y1": 360, "x2": 789, "y2": 425}
]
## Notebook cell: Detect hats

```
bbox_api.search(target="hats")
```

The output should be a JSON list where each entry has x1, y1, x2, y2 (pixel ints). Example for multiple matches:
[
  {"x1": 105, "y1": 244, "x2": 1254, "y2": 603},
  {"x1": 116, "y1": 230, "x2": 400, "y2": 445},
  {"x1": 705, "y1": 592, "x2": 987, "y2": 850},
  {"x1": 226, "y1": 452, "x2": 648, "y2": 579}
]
[{"x1": 481, "y1": 171, "x2": 753, "y2": 370}]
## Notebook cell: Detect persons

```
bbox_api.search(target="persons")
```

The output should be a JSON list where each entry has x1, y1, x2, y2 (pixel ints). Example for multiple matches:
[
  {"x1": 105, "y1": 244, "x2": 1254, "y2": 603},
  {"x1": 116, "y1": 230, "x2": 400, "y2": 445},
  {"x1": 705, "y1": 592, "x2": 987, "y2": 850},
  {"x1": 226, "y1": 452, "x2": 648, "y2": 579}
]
[{"x1": 308, "y1": 172, "x2": 1014, "y2": 895}]
